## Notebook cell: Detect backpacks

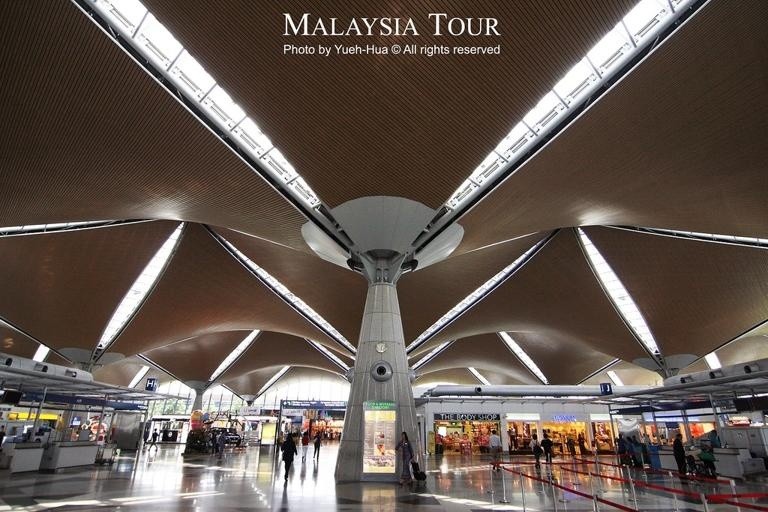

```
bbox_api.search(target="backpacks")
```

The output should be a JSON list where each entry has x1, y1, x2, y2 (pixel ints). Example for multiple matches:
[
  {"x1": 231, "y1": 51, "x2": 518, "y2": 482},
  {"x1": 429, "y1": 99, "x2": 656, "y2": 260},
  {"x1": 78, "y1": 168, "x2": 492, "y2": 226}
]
[{"x1": 531, "y1": 439, "x2": 544, "y2": 455}]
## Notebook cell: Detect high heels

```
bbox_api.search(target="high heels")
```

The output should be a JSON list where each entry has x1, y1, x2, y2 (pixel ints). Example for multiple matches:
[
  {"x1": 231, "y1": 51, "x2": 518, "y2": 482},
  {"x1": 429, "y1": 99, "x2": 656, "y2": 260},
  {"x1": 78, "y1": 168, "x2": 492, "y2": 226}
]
[{"x1": 397, "y1": 481, "x2": 413, "y2": 488}]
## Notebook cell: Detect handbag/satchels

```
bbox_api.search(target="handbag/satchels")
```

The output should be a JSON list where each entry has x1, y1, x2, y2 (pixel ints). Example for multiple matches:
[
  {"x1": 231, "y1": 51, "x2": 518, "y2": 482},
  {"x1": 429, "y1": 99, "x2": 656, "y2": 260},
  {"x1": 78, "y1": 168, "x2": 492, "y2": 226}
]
[{"x1": 411, "y1": 461, "x2": 420, "y2": 472}]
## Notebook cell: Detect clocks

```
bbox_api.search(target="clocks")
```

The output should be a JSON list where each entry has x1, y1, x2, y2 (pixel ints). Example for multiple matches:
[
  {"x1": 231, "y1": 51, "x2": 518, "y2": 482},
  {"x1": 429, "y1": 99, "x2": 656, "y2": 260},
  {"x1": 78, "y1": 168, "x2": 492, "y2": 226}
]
[{"x1": 375, "y1": 341, "x2": 389, "y2": 352}]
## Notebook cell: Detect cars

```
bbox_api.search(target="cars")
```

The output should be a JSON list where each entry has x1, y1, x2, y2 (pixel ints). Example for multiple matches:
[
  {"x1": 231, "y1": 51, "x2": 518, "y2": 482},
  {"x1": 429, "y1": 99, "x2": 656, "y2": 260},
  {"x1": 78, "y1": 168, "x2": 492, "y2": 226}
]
[
  {"x1": 23, "y1": 425, "x2": 61, "y2": 442},
  {"x1": 185, "y1": 427, "x2": 241, "y2": 452}
]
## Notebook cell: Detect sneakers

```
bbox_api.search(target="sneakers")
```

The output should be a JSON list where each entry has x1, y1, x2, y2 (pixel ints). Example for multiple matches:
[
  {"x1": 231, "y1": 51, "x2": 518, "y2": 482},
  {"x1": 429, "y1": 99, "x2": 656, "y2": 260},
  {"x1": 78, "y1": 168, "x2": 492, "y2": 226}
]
[{"x1": 492, "y1": 466, "x2": 501, "y2": 472}]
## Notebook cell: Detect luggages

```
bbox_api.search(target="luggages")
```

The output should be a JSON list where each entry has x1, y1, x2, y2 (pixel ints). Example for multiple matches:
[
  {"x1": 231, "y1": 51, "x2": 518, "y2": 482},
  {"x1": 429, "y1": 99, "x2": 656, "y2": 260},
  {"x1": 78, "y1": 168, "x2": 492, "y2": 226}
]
[{"x1": 413, "y1": 471, "x2": 427, "y2": 481}]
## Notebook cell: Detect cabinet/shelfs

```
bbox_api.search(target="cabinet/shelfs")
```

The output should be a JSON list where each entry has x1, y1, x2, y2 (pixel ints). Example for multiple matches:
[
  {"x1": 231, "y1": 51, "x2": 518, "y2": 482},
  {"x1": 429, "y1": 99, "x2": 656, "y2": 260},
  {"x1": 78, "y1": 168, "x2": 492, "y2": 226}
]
[
  {"x1": 1, "y1": 439, "x2": 117, "y2": 477},
  {"x1": 648, "y1": 427, "x2": 768, "y2": 480}
]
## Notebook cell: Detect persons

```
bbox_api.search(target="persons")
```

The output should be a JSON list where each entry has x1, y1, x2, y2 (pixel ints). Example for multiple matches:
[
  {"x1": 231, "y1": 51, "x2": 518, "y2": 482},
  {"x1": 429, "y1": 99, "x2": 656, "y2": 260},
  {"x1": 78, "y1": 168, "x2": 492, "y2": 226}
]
[
  {"x1": 78, "y1": 423, "x2": 90, "y2": 442},
  {"x1": 103, "y1": 430, "x2": 108, "y2": 443},
  {"x1": 672, "y1": 432, "x2": 689, "y2": 480},
  {"x1": 540, "y1": 433, "x2": 553, "y2": 465},
  {"x1": 487, "y1": 430, "x2": 501, "y2": 473},
  {"x1": 69, "y1": 425, "x2": 78, "y2": 442},
  {"x1": 526, "y1": 434, "x2": 541, "y2": 470},
  {"x1": 280, "y1": 433, "x2": 297, "y2": 482},
  {"x1": 707, "y1": 428, "x2": 721, "y2": 449},
  {"x1": 699, "y1": 444, "x2": 717, "y2": 477},
  {"x1": 0, "y1": 424, "x2": 7, "y2": 450},
  {"x1": 142, "y1": 426, "x2": 148, "y2": 448},
  {"x1": 33, "y1": 428, "x2": 44, "y2": 441},
  {"x1": 395, "y1": 431, "x2": 415, "y2": 488},
  {"x1": 563, "y1": 429, "x2": 664, "y2": 472},
  {"x1": 147, "y1": 428, "x2": 160, "y2": 452},
  {"x1": 278, "y1": 421, "x2": 341, "y2": 464},
  {"x1": 206, "y1": 429, "x2": 241, "y2": 460}
]
